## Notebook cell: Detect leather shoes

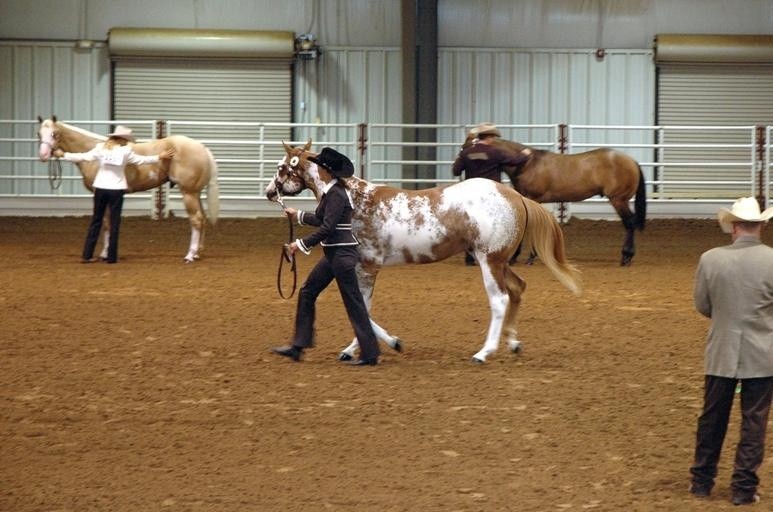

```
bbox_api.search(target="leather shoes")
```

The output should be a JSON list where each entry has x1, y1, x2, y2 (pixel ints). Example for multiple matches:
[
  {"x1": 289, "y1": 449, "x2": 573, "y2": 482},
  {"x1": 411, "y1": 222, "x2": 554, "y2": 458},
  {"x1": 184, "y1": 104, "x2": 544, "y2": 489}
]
[
  {"x1": 687, "y1": 479, "x2": 711, "y2": 497},
  {"x1": 270, "y1": 345, "x2": 301, "y2": 361},
  {"x1": 730, "y1": 491, "x2": 762, "y2": 507},
  {"x1": 349, "y1": 356, "x2": 378, "y2": 366}
]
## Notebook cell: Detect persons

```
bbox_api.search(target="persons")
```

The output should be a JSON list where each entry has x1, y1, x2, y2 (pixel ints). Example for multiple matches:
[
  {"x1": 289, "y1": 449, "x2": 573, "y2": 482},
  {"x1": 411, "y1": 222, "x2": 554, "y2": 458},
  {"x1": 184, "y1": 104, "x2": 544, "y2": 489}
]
[
  {"x1": 454, "y1": 123, "x2": 532, "y2": 265},
  {"x1": 271, "y1": 148, "x2": 381, "y2": 366},
  {"x1": 689, "y1": 197, "x2": 773, "y2": 505},
  {"x1": 53, "y1": 125, "x2": 176, "y2": 263}
]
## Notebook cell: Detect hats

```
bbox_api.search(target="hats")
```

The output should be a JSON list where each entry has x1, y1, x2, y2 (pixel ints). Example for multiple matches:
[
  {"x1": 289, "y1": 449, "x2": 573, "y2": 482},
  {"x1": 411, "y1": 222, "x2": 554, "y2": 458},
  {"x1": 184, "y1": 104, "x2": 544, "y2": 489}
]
[
  {"x1": 306, "y1": 147, "x2": 355, "y2": 192},
  {"x1": 717, "y1": 194, "x2": 773, "y2": 234},
  {"x1": 106, "y1": 124, "x2": 136, "y2": 143}
]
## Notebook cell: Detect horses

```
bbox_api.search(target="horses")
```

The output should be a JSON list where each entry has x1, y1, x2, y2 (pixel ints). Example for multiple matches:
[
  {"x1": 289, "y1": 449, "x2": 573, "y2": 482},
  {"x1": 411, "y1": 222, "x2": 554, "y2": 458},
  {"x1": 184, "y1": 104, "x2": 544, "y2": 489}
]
[
  {"x1": 451, "y1": 122, "x2": 648, "y2": 268},
  {"x1": 37, "y1": 112, "x2": 221, "y2": 265},
  {"x1": 264, "y1": 136, "x2": 582, "y2": 367}
]
